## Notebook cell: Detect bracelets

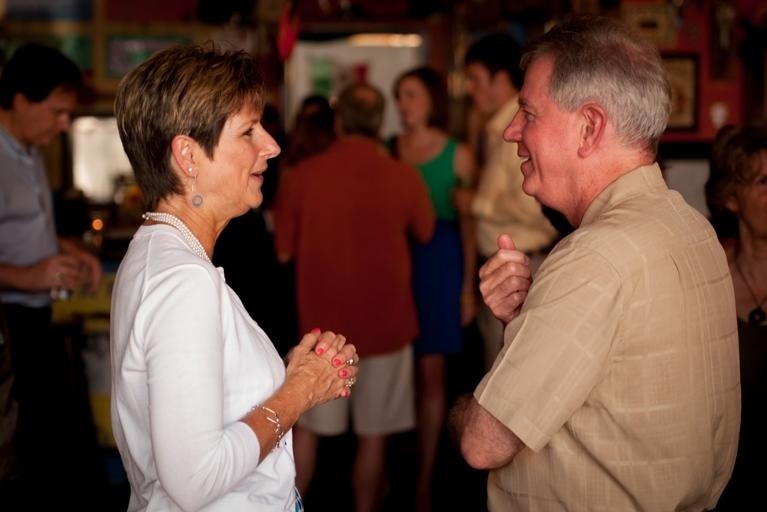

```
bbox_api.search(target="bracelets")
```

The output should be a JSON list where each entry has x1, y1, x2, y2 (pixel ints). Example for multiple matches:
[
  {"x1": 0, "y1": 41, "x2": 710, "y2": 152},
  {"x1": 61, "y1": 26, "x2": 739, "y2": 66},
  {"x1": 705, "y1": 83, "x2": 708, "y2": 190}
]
[{"x1": 252, "y1": 404, "x2": 284, "y2": 449}]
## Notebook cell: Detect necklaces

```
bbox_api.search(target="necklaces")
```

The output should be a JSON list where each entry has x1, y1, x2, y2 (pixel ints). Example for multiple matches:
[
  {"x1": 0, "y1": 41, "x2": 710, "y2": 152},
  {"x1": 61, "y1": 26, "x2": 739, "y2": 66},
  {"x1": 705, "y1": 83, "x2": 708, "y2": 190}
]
[{"x1": 140, "y1": 210, "x2": 212, "y2": 269}]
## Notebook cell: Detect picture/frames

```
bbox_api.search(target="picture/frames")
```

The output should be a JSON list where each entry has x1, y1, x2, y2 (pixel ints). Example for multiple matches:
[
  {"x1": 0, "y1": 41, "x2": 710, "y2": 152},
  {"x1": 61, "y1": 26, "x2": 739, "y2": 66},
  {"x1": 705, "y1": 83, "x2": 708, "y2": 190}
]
[{"x1": 658, "y1": 52, "x2": 698, "y2": 131}]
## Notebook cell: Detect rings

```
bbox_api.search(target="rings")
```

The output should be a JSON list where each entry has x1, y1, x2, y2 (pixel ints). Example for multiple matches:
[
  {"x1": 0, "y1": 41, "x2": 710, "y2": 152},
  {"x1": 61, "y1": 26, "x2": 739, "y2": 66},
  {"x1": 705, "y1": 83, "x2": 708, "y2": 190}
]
[
  {"x1": 345, "y1": 378, "x2": 352, "y2": 389},
  {"x1": 347, "y1": 359, "x2": 353, "y2": 365}
]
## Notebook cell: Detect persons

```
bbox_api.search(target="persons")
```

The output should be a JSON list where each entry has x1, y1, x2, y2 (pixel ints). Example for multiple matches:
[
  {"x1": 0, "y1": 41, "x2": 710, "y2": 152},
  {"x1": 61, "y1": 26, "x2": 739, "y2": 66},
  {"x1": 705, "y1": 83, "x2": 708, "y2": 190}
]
[
  {"x1": 103, "y1": 43, "x2": 359, "y2": 511},
  {"x1": 448, "y1": 34, "x2": 554, "y2": 388},
  {"x1": 703, "y1": 122, "x2": 766, "y2": 512},
  {"x1": 217, "y1": 64, "x2": 477, "y2": 510},
  {"x1": 1, "y1": 37, "x2": 104, "y2": 385},
  {"x1": 447, "y1": 15, "x2": 740, "y2": 511}
]
